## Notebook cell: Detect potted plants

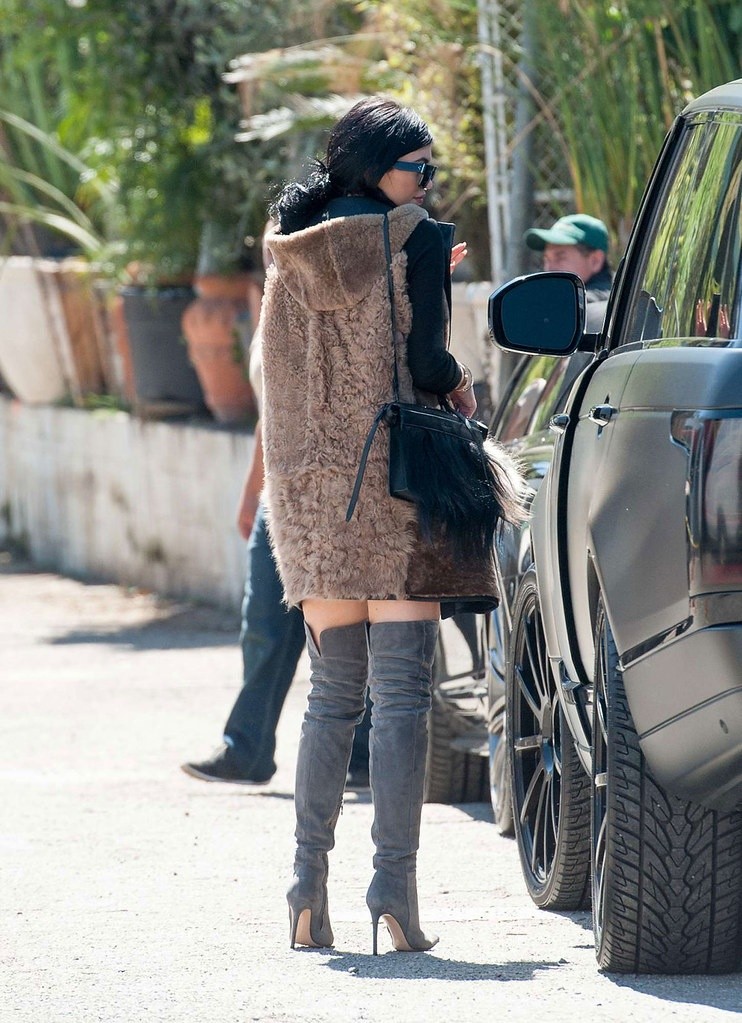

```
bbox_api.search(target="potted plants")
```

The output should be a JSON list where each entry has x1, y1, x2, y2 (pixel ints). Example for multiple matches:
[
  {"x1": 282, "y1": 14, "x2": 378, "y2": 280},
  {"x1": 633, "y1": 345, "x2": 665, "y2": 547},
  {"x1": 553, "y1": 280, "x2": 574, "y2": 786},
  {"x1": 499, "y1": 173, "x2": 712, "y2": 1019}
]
[{"x1": 1, "y1": 0, "x2": 286, "y2": 430}]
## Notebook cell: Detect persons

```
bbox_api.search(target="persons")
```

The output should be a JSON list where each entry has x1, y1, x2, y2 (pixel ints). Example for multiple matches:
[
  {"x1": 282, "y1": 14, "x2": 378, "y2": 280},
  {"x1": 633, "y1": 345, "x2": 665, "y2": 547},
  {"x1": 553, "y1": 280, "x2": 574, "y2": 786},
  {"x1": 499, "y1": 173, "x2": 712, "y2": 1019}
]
[
  {"x1": 694, "y1": 299, "x2": 731, "y2": 338},
  {"x1": 525, "y1": 213, "x2": 615, "y2": 303},
  {"x1": 261, "y1": 96, "x2": 477, "y2": 956},
  {"x1": 179, "y1": 216, "x2": 371, "y2": 792}
]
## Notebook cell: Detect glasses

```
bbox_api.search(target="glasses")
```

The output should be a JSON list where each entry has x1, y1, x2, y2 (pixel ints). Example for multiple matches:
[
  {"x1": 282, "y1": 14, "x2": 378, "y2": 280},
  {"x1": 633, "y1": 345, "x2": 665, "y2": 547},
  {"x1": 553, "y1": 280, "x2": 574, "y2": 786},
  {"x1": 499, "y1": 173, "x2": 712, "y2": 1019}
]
[{"x1": 393, "y1": 160, "x2": 437, "y2": 189}]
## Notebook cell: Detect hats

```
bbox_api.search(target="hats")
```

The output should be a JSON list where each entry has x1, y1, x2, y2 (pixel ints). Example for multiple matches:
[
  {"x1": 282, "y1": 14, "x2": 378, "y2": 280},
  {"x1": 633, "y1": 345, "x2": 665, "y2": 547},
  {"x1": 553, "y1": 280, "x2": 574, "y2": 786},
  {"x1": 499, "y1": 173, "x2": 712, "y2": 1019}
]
[{"x1": 526, "y1": 213, "x2": 608, "y2": 253}]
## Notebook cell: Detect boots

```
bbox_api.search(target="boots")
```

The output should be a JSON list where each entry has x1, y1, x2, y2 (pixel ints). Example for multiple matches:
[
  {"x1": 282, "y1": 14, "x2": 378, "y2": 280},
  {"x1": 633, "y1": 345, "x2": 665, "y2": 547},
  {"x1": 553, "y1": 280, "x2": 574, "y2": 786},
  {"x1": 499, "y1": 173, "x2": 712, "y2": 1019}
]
[
  {"x1": 363, "y1": 619, "x2": 438, "y2": 956},
  {"x1": 286, "y1": 622, "x2": 369, "y2": 948}
]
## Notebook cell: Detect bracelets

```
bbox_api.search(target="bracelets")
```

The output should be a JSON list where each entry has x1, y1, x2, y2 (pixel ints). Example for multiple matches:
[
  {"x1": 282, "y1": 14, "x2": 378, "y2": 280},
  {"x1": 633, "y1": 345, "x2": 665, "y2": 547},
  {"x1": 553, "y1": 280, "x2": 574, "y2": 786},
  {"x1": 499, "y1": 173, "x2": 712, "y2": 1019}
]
[{"x1": 455, "y1": 361, "x2": 473, "y2": 392}]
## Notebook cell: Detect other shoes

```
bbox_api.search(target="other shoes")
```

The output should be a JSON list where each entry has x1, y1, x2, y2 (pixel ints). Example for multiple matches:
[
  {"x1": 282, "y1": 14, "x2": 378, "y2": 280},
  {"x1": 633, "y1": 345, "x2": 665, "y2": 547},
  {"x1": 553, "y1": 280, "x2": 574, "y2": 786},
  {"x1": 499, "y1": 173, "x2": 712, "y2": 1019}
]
[
  {"x1": 182, "y1": 748, "x2": 278, "y2": 784},
  {"x1": 344, "y1": 765, "x2": 372, "y2": 792}
]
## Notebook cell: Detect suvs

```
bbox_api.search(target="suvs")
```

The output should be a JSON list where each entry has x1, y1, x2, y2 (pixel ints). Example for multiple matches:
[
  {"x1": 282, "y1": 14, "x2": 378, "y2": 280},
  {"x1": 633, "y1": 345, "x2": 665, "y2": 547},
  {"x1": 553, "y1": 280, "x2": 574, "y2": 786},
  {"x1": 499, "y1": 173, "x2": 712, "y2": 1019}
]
[{"x1": 505, "y1": 71, "x2": 741, "y2": 981}]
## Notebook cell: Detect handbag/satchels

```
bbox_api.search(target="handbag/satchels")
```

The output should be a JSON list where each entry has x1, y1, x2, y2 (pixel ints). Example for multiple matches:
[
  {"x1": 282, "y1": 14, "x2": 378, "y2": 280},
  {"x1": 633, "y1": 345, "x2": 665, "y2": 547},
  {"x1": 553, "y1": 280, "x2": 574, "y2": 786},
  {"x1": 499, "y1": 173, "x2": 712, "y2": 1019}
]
[{"x1": 380, "y1": 401, "x2": 500, "y2": 523}]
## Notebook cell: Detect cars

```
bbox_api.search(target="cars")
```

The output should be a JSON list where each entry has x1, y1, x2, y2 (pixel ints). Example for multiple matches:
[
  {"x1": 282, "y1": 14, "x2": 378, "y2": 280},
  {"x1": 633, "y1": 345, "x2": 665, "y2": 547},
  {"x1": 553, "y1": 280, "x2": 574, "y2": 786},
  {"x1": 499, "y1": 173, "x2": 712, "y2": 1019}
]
[{"x1": 422, "y1": 299, "x2": 613, "y2": 841}]
